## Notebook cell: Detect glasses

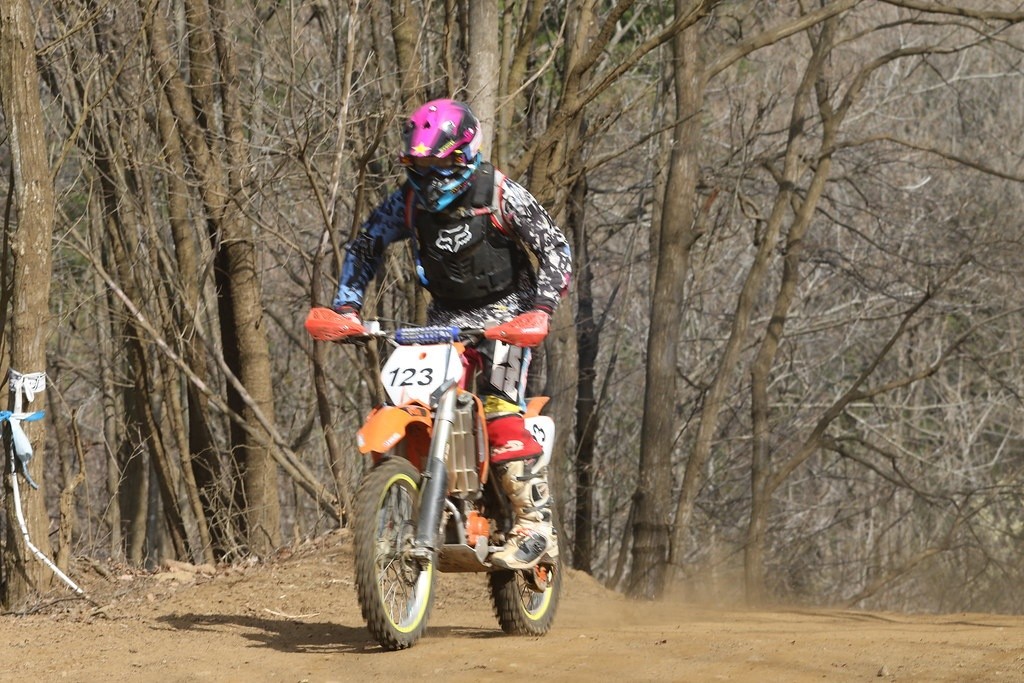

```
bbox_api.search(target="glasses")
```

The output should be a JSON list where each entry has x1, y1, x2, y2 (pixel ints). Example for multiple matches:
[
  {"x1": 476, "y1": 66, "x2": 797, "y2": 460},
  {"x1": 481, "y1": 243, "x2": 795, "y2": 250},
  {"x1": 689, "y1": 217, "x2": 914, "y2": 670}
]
[{"x1": 399, "y1": 147, "x2": 468, "y2": 180}]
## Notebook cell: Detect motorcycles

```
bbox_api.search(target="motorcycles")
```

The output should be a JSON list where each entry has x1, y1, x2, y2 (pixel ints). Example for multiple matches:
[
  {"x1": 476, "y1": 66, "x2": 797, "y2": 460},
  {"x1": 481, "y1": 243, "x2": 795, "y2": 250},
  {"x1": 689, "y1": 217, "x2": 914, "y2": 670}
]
[{"x1": 304, "y1": 308, "x2": 562, "y2": 652}]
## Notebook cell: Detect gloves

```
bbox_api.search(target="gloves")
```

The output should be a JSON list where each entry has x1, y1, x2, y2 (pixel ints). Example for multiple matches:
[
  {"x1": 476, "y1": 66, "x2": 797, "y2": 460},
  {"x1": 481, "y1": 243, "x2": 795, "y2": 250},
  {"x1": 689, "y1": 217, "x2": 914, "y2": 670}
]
[
  {"x1": 520, "y1": 303, "x2": 553, "y2": 339},
  {"x1": 331, "y1": 300, "x2": 369, "y2": 348}
]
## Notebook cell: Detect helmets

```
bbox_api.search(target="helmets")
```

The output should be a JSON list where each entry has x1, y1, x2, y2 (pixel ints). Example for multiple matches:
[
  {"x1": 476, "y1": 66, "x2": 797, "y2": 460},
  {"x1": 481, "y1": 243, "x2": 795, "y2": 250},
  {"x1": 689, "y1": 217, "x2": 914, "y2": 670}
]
[{"x1": 398, "y1": 98, "x2": 482, "y2": 214}]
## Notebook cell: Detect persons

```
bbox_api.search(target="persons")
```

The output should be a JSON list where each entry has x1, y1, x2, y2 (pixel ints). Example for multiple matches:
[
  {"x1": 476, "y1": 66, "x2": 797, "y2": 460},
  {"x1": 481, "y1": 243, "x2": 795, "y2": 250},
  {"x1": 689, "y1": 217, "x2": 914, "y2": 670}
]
[{"x1": 333, "y1": 99, "x2": 572, "y2": 571}]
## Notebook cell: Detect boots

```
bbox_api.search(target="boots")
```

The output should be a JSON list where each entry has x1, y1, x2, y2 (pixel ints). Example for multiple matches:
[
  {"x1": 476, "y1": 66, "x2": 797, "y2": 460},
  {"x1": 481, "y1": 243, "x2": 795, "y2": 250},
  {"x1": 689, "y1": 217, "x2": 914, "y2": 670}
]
[{"x1": 489, "y1": 455, "x2": 560, "y2": 570}]
